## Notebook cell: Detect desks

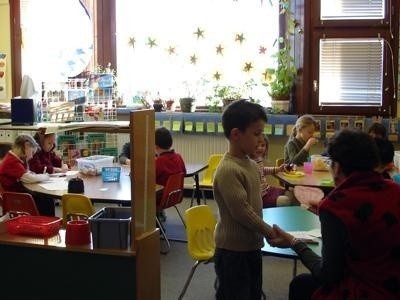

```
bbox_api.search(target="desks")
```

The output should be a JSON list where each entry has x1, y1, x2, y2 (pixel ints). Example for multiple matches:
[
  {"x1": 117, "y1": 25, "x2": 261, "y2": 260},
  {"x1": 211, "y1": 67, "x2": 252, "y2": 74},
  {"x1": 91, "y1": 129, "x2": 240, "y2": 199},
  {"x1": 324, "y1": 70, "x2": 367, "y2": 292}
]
[
  {"x1": 269, "y1": 162, "x2": 341, "y2": 198},
  {"x1": 18, "y1": 158, "x2": 208, "y2": 255},
  {"x1": 184, "y1": 164, "x2": 209, "y2": 205},
  {"x1": 1, "y1": 119, "x2": 130, "y2": 145},
  {"x1": 259, "y1": 205, "x2": 323, "y2": 300}
]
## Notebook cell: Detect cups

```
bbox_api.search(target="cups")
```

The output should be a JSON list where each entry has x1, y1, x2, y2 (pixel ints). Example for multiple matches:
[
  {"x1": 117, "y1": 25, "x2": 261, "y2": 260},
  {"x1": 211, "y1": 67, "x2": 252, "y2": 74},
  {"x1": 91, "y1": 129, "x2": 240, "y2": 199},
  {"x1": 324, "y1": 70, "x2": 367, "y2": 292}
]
[{"x1": 303, "y1": 162, "x2": 314, "y2": 177}]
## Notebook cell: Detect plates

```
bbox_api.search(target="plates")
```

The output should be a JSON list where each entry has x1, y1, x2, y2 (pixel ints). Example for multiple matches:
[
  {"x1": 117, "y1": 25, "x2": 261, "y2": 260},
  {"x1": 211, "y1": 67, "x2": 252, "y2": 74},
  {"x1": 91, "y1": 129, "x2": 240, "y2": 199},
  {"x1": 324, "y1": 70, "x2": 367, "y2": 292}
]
[
  {"x1": 284, "y1": 170, "x2": 303, "y2": 178},
  {"x1": 320, "y1": 179, "x2": 334, "y2": 187}
]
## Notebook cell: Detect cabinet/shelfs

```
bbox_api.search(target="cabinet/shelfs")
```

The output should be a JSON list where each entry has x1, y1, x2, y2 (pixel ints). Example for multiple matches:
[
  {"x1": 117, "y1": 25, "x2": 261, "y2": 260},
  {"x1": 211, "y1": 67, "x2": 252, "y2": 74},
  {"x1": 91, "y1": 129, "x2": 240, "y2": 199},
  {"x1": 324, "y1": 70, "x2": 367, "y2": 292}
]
[{"x1": 0, "y1": 108, "x2": 160, "y2": 299}]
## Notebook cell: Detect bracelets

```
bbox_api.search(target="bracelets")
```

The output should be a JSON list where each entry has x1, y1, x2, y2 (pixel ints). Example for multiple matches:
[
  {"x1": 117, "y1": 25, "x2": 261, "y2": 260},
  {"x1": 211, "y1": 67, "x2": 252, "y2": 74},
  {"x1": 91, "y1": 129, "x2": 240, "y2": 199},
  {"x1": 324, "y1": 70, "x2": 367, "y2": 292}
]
[{"x1": 290, "y1": 237, "x2": 297, "y2": 247}]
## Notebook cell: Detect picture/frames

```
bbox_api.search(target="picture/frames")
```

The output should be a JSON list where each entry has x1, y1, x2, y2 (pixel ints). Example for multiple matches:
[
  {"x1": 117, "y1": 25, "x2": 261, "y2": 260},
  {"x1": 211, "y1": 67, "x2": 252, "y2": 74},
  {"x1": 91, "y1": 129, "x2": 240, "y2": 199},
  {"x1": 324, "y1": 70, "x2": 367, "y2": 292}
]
[{"x1": 315, "y1": 120, "x2": 399, "y2": 135}]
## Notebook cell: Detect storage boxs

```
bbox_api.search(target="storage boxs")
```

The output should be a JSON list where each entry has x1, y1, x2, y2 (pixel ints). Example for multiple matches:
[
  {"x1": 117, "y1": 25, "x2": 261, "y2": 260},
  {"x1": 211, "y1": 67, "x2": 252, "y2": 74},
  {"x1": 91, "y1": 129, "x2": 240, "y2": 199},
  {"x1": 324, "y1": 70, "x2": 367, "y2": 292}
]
[{"x1": 86, "y1": 206, "x2": 133, "y2": 251}]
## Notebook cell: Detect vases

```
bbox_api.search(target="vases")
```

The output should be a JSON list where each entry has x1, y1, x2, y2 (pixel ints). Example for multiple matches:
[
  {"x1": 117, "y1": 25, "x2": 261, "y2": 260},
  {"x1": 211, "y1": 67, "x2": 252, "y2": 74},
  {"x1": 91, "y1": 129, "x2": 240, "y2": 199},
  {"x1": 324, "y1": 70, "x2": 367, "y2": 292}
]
[{"x1": 179, "y1": 98, "x2": 196, "y2": 113}]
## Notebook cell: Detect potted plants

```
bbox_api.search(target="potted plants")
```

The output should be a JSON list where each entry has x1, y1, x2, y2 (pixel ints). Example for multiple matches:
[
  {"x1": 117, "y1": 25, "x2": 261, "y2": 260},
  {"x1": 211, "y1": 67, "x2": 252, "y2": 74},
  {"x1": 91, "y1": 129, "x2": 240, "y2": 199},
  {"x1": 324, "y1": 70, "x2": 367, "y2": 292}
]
[
  {"x1": 266, "y1": 38, "x2": 297, "y2": 114},
  {"x1": 153, "y1": 99, "x2": 163, "y2": 112},
  {"x1": 219, "y1": 87, "x2": 240, "y2": 107}
]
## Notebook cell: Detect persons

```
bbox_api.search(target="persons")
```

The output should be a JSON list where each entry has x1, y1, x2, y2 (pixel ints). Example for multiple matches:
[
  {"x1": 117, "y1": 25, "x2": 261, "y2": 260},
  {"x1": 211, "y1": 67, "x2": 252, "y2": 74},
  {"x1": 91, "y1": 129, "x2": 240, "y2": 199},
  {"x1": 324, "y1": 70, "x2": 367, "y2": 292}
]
[
  {"x1": 0, "y1": 134, "x2": 48, "y2": 199},
  {"x1": 368, "y1": 123, "x2": 386, "y2": 139},
  {"x1": 154, "y1": 127, "x2": 186, "y2": 238},
  {"x1": 283, "y1": 115, "x2": 318, "y2": 164},
  {"x1": 215, "y1": 100, "x2": 277, "y2": 299},
  {"x1": 119, "y1": 133, "x2": 130, "y2": 164},
  {"x1": 29, "y1": 128, "x2": 72, "y2": 174},
  {"x1": 269, "y1": 129, "x2": 399, "y2": 299},
  {"x1": 375, "y1": 138, "x2": 394, "y2": 178},
  {"x1": 251, "y1": 135, "x2": 285, "y2": 206}
]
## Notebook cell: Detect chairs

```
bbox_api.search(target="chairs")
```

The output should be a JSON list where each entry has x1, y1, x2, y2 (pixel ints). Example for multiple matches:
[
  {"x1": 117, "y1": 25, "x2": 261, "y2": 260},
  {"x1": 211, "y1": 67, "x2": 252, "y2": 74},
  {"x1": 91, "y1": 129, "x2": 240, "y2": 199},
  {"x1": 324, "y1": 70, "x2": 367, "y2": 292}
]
[
  {"x1": 178, "y1": 205, "x2": 269, "y2": 300},
  {"x1": 190, "y1": 152, "x2": 232, "y2": 209},
  {"x1": 61, "y1": 193, "x2": 96, "y2": 229},
  {"x1": 154, "y1": 170, "x2": 190, "y2": 255},
  {"x1": 2, "y1": 191, "x2": 44, "y2": 217}
]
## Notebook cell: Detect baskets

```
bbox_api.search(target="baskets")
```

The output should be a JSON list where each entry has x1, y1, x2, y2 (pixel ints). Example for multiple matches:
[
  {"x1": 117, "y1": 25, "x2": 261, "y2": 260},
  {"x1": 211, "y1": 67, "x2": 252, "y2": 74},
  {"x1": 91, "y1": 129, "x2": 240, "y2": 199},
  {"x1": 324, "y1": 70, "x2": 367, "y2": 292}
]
[{"x1": 102, "y1": 166, "x2": 121, "y2": 183}]
